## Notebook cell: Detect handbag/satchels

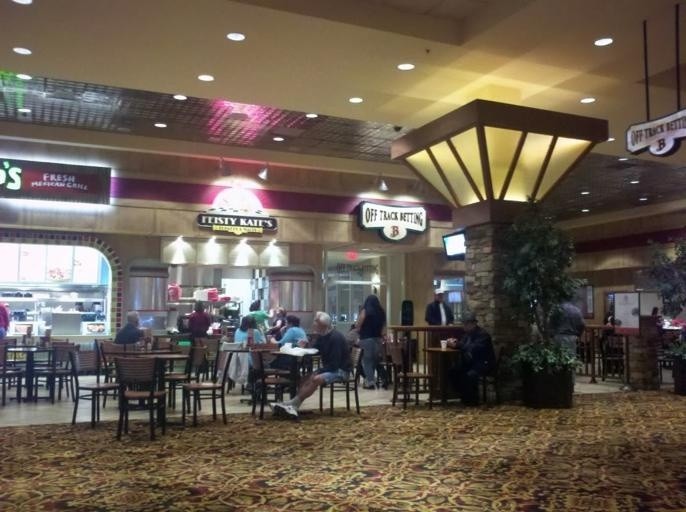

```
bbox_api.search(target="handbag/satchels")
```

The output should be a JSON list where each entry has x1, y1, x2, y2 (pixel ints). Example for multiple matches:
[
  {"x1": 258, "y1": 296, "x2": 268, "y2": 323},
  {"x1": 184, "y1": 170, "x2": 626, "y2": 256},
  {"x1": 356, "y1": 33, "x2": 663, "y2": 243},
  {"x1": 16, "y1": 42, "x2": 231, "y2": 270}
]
[{"x1": 373, "y1": 342, "x2": 385, "y2": 362}]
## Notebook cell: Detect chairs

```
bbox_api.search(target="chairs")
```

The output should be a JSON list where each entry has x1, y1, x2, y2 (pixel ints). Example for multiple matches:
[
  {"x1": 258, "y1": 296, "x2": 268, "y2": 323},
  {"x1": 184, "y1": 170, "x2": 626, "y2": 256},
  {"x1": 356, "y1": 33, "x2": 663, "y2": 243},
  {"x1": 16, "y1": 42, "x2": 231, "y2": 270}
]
[{"x1": 579, "y1": 322, "x2": 628, "y2": 388}]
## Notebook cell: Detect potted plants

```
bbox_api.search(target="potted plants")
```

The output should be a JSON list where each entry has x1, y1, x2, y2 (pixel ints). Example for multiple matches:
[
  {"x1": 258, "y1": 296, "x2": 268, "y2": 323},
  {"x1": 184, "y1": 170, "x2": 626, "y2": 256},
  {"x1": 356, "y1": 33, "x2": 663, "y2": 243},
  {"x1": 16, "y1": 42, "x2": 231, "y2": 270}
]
[
  {"x1": 500, "y1": 195, "x2": 591, "y2": 409},
  {"x1": 646, "y1": 235, "x2": 686, "y2": 395}
]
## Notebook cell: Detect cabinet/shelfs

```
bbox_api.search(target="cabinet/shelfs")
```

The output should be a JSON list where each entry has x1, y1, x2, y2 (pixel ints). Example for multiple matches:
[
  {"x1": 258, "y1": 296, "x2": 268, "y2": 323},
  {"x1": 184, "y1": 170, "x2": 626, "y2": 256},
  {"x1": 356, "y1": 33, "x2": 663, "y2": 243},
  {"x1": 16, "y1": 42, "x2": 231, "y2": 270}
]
[{"x1": 0, "y1": 243, "x2": 101, "y2": 289}]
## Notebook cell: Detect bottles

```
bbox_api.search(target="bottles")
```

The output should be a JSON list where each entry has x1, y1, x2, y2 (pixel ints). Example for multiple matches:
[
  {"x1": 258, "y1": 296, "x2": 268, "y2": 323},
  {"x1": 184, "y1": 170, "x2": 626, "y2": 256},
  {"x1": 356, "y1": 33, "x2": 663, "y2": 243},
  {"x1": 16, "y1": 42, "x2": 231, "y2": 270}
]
[{"x1": 74, "y1": 302, "x2": 101, "y2": 312}]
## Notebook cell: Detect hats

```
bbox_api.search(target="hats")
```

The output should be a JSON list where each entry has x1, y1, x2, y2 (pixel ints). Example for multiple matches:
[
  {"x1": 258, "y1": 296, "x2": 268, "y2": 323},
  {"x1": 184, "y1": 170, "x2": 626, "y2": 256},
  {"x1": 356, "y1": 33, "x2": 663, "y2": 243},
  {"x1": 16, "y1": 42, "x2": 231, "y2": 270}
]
[
  {"x1": 434, "y1": 288, "x2": 445, "y2": 296},
  {"x1": 460, "y1": 313, "x2": 477, "y2": 323}
]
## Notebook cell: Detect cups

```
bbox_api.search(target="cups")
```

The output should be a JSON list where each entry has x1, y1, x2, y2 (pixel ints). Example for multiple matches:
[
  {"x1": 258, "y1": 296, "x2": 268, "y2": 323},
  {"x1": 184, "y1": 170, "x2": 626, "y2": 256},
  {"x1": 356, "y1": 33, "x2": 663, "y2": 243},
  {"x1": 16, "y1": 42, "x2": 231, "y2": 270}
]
[
  {"x1": 439, "y1": 340, "x2": 447, "y2": 350},
  {"x1": 212, "y1": 328, "x2": 220, "y2": 334},
  {"x1": 284, "y1": 343, "x2": 292, "y2": 349}
]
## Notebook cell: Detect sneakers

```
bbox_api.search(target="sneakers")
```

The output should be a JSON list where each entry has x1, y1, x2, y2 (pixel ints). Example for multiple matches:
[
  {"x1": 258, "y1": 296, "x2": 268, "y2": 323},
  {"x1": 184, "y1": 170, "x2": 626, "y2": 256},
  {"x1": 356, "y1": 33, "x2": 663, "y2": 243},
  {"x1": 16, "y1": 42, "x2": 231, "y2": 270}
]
[{"x1": 269, "y1": 402, "x2": 298, "y2": 420}]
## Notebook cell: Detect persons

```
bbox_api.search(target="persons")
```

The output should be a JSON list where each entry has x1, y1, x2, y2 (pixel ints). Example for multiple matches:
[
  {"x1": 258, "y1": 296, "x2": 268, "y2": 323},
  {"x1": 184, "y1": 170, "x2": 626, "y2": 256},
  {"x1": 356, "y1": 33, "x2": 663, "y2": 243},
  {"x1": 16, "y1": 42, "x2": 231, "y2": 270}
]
[
  {"x1": 0, "y1": 299, "x2": 11, "y2": 340},
  {"x1": 446, "y1": 311, "x2": 498, "y2": 406},
  {"x1": 110, "y1": 289, "x2": 387, "y2": 419},
  {"x1": 592, "y1": 306, "x2": 685, "y2": 372},
  {"x1": 548, "y1": 301, "x2": 585, "y2": 354},
  {"x1": 424, "y1": 288, "x2": 453, "y2": 327}
]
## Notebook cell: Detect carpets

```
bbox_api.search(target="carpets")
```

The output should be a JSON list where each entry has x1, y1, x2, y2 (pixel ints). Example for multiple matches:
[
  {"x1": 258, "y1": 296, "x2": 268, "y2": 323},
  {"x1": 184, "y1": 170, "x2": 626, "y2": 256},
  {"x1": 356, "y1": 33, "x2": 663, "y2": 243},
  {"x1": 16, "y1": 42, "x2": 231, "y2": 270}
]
[{"x1": 1, "y1": 388, "x2": 686, "y2": 511}]
